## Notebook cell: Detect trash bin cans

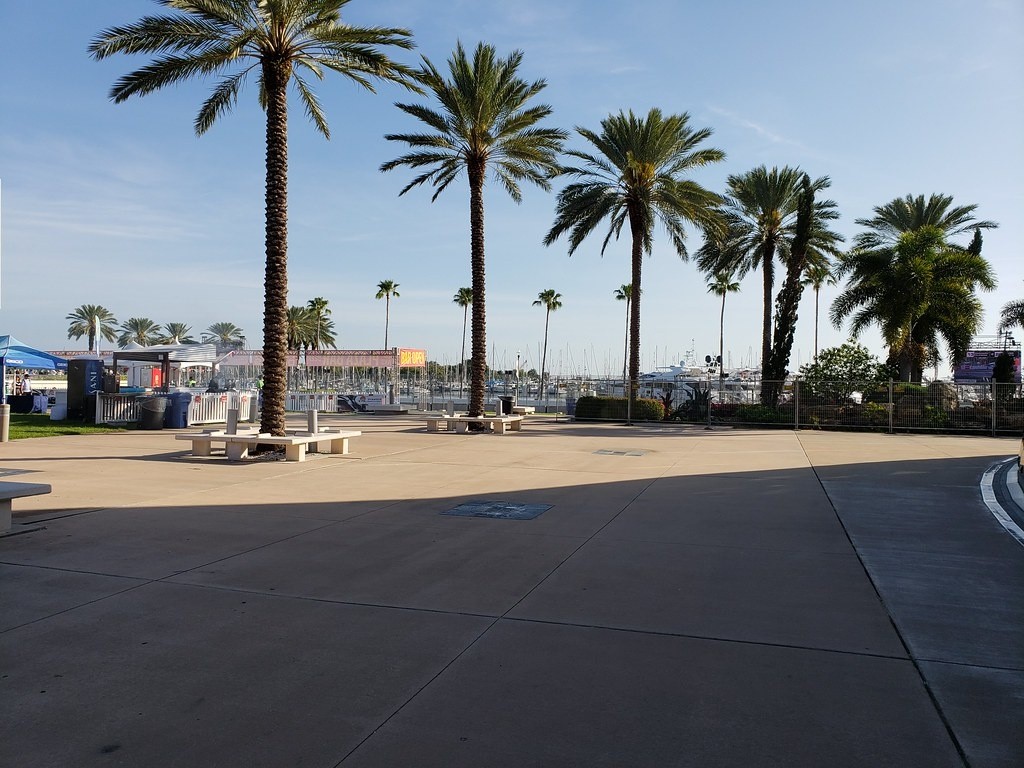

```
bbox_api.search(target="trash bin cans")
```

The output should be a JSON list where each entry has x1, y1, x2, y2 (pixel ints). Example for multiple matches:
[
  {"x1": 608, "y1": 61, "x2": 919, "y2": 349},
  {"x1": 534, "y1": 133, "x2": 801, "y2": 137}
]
[
  {"x1": 159, "y1": 393, "x2": 192, "y2": 429},
  {"x1": 136, "y1": 395, "x2": 169, "y2": 430},
  {"x1": 498, "y1": 395, "x2": 515, "y2": 414}
]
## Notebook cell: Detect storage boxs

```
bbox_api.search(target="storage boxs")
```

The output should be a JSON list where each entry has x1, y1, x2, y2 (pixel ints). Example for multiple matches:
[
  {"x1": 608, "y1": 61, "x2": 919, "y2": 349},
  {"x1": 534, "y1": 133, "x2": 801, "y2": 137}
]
[{"x1": 104, "y1": 374, "x2": 120, "y2": 393}]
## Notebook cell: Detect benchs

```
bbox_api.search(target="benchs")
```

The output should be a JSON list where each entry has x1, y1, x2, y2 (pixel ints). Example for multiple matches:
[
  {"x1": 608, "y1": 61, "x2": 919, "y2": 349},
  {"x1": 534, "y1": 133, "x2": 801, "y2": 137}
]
[
  {"x1": 175, "y1": 427, "x2": 361, "y2": 461},
  {"x1": 0, "y1": 481, "x2": 51, "y2": 533},
  {"x1": 418, "y1": 414, "x2": 523, "y2": 434}
]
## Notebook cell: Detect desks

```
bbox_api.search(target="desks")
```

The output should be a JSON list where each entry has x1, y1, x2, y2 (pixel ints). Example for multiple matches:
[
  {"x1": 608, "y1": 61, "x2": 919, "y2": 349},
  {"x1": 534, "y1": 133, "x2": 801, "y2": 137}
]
[
  {"x1": 513, "y1": 406, "x2": 535, "y2": 415},
  {"x1": 5, "y1": 394, "x2": 48, "y2": 414}
]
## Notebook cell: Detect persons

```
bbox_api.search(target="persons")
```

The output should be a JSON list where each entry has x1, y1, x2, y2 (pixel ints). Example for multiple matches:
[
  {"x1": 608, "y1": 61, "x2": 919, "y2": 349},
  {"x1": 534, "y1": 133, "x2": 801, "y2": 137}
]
[
  {"x1": 206, "y1": 372, "x2": 223, "y2": 393},
  {"x1": 187, "y1": 376, "x2": 197, "y2": 388},
  {"x1": 20, "y1": 374, "x2": 32, "y2": 396},
  {"x1": 6, "y1": 366, "x2": 66, "y2": 375},
  {"x1": 255, "y1": 375, "x2": 264, "y2": 390},
  {"x1": 11, "y1": 376, "x2": 22, "y2": 395}
]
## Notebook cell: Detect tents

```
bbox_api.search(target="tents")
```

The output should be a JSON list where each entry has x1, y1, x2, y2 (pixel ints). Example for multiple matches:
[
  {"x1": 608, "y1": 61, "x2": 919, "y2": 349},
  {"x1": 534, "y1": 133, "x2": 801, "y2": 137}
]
[
  {"x1": 0, "y1": 334, "x2": 68, "y2": 406},
  {"x1": 101, "y1": 338, "x2": 218, "y2": 388}
]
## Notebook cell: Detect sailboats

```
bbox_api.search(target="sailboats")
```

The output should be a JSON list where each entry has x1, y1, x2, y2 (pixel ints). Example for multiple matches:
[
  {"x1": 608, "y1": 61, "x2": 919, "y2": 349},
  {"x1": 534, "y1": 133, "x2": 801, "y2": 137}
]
[{"x1": 232, "y1": 341, "x2": 764, "y2": 404}]
